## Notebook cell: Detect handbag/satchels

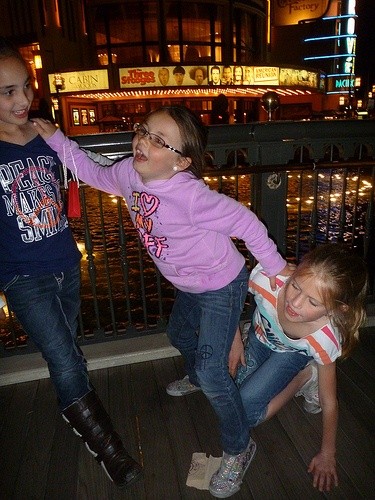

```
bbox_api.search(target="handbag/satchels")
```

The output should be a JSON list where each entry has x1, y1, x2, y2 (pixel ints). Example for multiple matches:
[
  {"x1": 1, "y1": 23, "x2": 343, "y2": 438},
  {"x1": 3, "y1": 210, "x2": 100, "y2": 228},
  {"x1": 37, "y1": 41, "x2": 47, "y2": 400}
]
[{"x1": 63, "y1": 139, "x2": 82, "y2": 218}]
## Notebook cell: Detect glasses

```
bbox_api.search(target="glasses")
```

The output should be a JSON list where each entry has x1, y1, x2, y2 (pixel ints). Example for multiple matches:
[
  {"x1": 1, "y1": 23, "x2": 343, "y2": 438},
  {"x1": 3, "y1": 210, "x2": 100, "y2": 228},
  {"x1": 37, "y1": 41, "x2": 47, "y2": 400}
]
[{"x1": 133, "y1": 122, "x2": 184, "y2": 157}]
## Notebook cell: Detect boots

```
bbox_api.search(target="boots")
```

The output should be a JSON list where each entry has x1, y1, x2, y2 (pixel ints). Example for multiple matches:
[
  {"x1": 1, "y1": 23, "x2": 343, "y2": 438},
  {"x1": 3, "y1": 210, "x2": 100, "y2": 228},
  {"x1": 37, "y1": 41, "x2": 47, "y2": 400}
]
[{"x1": 60, "y1": 389, "x2": 143, "y2": 490}]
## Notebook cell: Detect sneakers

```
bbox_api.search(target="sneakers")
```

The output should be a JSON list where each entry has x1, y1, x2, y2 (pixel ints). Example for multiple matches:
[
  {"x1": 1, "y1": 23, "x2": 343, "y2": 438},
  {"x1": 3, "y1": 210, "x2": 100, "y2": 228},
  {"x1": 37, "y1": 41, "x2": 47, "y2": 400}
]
[
  {"x1": 295, "y1": 360, "x2": 322, "y2": 414},
  {"x1": 166, "y1": 374, "x2": 201, "y2": 396},
  {"x1": 209, "y1": 437, "x2": 257, "y2": 498}
]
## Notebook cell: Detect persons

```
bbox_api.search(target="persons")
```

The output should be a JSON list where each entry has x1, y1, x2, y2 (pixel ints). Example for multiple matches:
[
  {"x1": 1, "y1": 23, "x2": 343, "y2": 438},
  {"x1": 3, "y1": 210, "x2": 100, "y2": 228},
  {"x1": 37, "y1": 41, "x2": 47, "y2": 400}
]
[
  {"x1": 0, "y1": 44, "x2": 143, "y2": 488},
  {"x1": 228, "y1": 240, "x2": 368, "y2": 492},
  {"x1": 158, "y1": 66, "x2": 250, "y2": 85},
  {"x1": 30, "y1": 104, "x2": 296, "y2": 497}
]
